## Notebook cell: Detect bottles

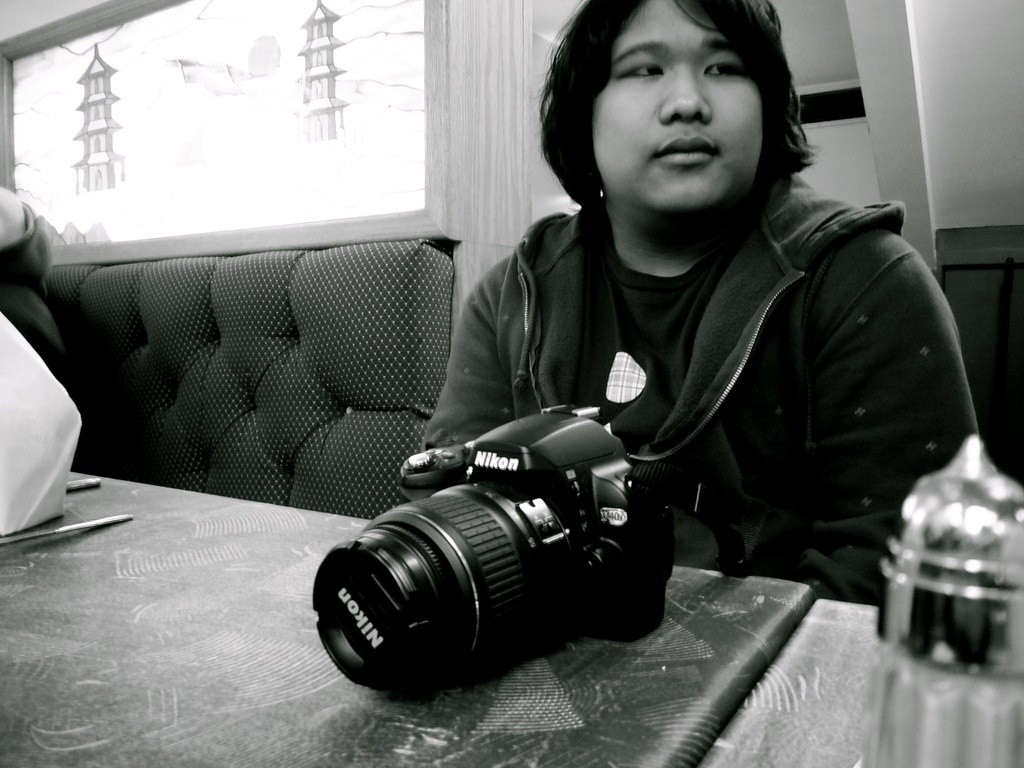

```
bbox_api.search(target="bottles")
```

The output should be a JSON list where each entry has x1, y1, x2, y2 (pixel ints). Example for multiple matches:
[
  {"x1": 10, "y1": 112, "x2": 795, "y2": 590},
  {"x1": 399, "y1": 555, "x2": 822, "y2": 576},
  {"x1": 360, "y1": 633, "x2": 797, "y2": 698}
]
[{"x1": 851, "y1": 436, "x2": 1024, "y2": 768}]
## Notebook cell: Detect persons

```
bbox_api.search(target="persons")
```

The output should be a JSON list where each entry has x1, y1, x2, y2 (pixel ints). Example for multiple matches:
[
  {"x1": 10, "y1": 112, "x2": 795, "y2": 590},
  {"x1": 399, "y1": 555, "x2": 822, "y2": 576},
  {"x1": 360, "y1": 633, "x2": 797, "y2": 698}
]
[{"x1": 394, "y1": 0, "x2": 982, "y2": 606}]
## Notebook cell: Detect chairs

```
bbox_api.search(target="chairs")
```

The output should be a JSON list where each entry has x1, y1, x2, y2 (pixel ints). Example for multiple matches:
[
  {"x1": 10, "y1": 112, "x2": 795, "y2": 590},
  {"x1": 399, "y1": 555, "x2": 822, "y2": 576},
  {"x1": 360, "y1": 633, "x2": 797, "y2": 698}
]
[{"x1": 34, "y1": 230, "x2": 453, "y2": 518}]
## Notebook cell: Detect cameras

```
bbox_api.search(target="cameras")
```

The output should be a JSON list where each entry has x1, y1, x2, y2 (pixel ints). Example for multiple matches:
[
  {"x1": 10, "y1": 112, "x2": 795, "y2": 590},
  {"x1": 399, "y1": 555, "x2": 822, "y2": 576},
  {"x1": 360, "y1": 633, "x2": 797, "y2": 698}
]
[{"x1": 313, "y1": 410, "x2": 676, "y2": 695}]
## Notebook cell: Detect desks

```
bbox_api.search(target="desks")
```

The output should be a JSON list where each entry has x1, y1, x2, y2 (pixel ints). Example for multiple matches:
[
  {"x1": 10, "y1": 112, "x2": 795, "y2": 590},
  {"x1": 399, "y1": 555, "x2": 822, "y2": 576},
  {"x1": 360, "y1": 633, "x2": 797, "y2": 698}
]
[{"x1": 0, "y1": 470, "x2": 1024, "y2": 768}]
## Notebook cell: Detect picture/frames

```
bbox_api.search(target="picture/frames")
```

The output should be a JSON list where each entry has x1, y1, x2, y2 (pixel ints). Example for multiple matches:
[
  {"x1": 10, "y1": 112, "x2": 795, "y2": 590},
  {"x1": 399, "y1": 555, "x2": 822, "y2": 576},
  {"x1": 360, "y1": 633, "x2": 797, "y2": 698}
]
[{"x1": 0, "y1": 0, "x2": 454, "y2": 268}]
